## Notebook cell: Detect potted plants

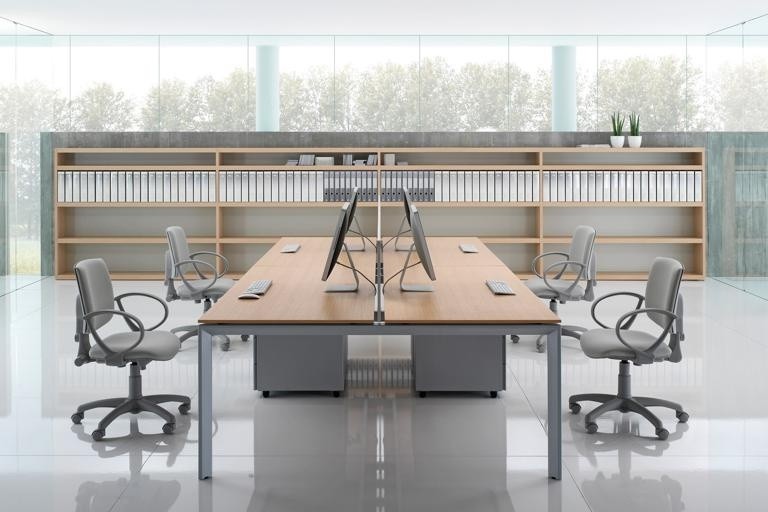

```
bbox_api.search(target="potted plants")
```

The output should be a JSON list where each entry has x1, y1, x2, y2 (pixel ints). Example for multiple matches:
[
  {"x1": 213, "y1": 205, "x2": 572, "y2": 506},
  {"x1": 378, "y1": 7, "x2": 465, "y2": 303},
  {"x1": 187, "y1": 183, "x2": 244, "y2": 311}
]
[{"x1": 609, "y1": 111, "x2": 642, "y2": 147}]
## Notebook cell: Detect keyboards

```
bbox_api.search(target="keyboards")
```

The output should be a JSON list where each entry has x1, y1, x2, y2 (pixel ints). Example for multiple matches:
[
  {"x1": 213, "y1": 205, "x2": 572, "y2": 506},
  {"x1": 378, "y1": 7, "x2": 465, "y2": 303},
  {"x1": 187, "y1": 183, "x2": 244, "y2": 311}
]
[
  {"x1": 281, "y1": 243, "x2": 300, "y2": 253},
  {"x1": 247, "y1": 279, "x2": 272, "y2": 294},
  {"x1": 485, "y1": 278, "x2": 518, "y2": 298},
  {"x1": 458, "y1": 242, "x2": 478, "y2": 253}
]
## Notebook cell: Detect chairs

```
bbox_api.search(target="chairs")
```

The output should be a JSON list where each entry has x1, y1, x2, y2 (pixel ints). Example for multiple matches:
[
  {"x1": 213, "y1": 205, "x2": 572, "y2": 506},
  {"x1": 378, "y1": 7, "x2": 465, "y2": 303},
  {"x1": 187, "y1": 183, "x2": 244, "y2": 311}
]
[
  {"x1": 71, "y1": 225, "x2": 250, "y2": 442},
  {"x1": 510, "y1": 224, "x2": 690, "y2": 440}
]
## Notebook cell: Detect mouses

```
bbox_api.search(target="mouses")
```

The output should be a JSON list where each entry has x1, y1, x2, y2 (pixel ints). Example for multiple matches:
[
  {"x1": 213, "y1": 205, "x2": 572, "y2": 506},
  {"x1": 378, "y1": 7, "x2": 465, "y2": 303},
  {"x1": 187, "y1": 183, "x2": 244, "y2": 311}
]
[{"x1": 238, "y1": 292, "x2": 259, "y2": 299}]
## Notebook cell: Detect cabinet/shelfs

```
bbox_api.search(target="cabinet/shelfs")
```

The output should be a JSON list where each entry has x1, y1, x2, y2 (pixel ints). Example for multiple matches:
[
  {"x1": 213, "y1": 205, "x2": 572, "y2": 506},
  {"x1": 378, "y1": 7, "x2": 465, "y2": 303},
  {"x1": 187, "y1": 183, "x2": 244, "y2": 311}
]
[{"x1": 52, "y1": 147, "x2": 709, "y2": 282}]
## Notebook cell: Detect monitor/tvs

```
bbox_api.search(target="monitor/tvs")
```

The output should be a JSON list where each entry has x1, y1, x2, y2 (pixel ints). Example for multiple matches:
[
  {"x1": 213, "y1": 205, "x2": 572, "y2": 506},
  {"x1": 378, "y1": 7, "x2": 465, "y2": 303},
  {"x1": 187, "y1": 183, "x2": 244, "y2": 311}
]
[
  {"x1": 399, "y1": 204, "x2": 436, "y2": 293},
  {"x1": 341, "y1": 187, "x2": 366, "y2": 253},
  {"x1": 322, "y1": 203, "x2": 359, "y2": 292},
  {"x1": 394, "y1": 186, "x2": 437, "y2": 250}
]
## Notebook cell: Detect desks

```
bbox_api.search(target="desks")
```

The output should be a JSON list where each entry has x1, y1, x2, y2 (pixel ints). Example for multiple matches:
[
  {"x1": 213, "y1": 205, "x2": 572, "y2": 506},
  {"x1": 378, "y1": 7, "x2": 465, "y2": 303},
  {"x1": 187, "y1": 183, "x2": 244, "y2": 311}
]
[{"x1": 197, "y1": 235, "x2": 563, "y2": 481}]
